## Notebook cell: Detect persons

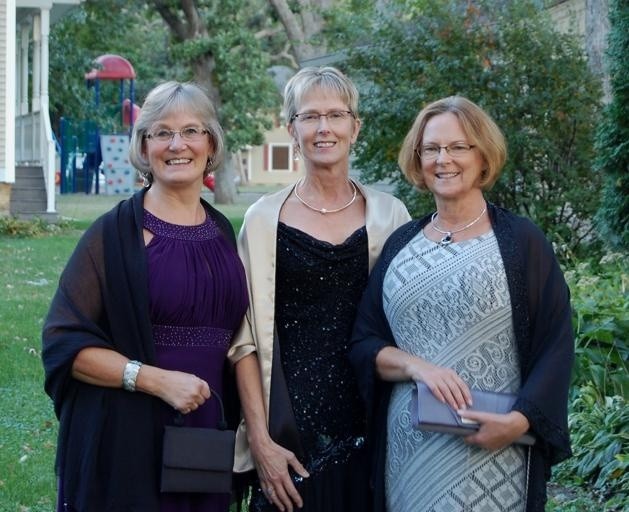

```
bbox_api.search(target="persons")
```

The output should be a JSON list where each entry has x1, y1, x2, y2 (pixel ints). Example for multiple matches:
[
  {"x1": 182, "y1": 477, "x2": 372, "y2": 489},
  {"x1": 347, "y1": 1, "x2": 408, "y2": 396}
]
[
  {"x1": 351, "y1": 96, "x2": 575, "y2": 512},
  {"x1": 42, "y1": 81, "x2": 248, "y2": 512},
  {"x1": 225, "y1": 65, "x2": 412, "y2": 512}
]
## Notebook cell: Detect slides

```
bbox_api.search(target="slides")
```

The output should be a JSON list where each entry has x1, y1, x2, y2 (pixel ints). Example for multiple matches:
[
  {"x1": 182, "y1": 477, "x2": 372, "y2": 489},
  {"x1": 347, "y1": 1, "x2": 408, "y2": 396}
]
[{"x1": 122, "y1": 98, "x2": 215, "y2": 192}]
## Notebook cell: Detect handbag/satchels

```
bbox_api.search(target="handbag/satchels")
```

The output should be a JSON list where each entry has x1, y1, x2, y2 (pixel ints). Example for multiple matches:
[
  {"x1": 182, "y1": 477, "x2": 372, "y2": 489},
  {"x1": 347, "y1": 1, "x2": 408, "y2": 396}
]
[
  {"x1": 409, "y1": 380, "x2": 537, "y2": 449},
  {"x1": 158, "y1": 425, "x2": 236, "y2": 496}
]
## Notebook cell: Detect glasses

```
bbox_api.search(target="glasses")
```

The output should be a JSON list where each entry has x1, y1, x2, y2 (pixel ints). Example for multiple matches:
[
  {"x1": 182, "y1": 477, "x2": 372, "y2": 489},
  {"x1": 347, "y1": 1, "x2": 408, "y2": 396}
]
[
  {"x1": 292, "y1": 110, "x2": 352, "y2": 126},
  {"x1": 414, "y1": 141, "x2": 475, "y2": 161},
  {"x1": 142, "y1": 126, "x2": 209, "y2": 144}
]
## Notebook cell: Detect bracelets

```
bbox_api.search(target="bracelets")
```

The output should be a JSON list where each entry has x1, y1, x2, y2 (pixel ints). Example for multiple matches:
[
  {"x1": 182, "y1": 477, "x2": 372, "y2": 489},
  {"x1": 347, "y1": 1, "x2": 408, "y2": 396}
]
[{"x1": 123, "y1": 360, "x2": 143, "y2": 392}]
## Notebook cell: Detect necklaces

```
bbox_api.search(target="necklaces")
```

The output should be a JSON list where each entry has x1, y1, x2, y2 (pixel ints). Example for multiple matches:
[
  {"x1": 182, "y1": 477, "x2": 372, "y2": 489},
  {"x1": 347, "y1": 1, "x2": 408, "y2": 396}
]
[
  {"x1": 431, "y1": 203, "x2": 486, "y2": 245},
  {"x1": 294, "y1": 177, "x2": 356, "y2": 214}
]
